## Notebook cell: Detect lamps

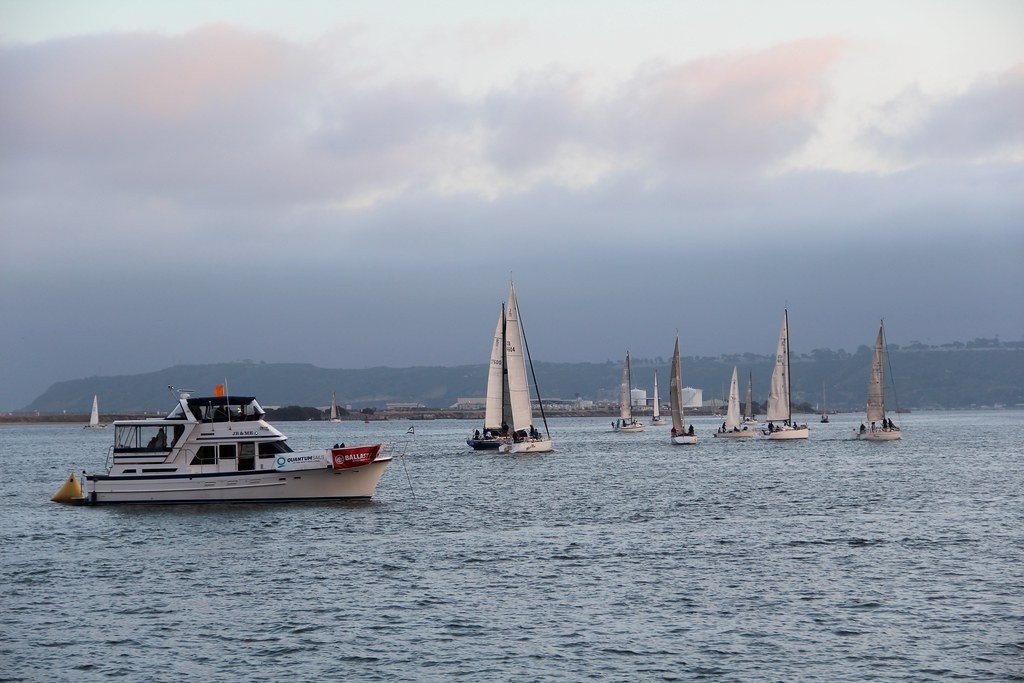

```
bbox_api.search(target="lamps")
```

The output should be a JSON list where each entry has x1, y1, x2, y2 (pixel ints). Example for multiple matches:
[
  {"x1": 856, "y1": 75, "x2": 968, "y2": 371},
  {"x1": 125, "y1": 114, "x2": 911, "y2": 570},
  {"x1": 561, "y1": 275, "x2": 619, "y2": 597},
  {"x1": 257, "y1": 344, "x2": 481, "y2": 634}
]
[
  {"x1": 168, "y1": 385, "x2": 174, "y2": 391},
  {"x1": 255, "y1": 431, "x2": 258, "y2": 435}
]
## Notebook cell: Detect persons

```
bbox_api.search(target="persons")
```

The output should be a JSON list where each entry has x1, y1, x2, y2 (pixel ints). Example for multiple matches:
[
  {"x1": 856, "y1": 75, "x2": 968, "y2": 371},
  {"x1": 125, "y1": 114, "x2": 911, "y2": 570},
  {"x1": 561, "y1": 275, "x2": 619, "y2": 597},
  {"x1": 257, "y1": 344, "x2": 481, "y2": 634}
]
[
  {"x1": 671, "y1": 427, "x2": 677, "y2": 437},
  {"x1": 688, "y1": 424, "x2": 695, "y2": 435},
  {"x1": 485, "y1": 431, "x2": 493, "y2": 440},
  {"x1": 502, "y1": 421, "x2": 509, "y2": 433},
  {"x1": 860, "y1": 424, "x2": 865, "y2": 430},
  {"x1": 612, "y1": 419, "x2": 626, "y2": 428},
  {"x1": 734, "y1": 427, "x2": 739, "y2": 432},
  {"x1": 718, "y1": 422, "x2": 726, "y2": 433},
  {"x1": 768, "y1": 421, "x2": 797, "y2": 432},
  {"x1": 529, "y1": 425, "x2": 543, "y2": 438},
  {"x1": 681, "y1": 426, "x2": 686, "y2": 433},
  {"x1": 883, "y1": 417, "x2": 892, "y2": 429}
]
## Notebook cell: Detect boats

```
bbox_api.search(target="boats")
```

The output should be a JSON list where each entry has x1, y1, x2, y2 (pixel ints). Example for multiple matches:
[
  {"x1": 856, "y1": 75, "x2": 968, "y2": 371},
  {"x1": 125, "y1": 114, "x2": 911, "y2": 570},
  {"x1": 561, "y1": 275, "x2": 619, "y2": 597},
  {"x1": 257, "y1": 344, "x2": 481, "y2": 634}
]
[{"x1": 47, "y1": 376, "x2": 408, "y2": 506}]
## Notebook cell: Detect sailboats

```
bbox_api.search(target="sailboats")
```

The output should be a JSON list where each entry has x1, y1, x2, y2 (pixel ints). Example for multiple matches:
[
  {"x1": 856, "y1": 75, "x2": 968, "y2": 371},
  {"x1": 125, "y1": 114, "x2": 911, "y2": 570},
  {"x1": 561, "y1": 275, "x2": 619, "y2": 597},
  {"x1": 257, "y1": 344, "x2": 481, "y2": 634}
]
[
  {"x1": 759, "y1": 306, "x2": 813, "y2": 440},
  {"x1": 462, "y1": 268, "x2": 556, "y2": 456},
  {"x1": 712, "y1": 364, "x2": 758, "y2": 437},
  {"x1": 821, "y1": 383, "x2": 830, "y2": 423},
  {"x1": 609, "y1": 350, "x2": 647, "y2": 433},
  {"x1": 647, "y1": 369, "x2": 670, "y2": 425},
  {"x1": 740, "y1": 368, "x2": 758, "y2": 425},
  {"x1": 854, "y1": 318, "x2": 903, "y2": 441},
  {"x1": 669, "y1": 336, "x2": 706, "y2": 445},
  {"x1": 328, "y1": 391, "x2": 341, "y2": 423}
]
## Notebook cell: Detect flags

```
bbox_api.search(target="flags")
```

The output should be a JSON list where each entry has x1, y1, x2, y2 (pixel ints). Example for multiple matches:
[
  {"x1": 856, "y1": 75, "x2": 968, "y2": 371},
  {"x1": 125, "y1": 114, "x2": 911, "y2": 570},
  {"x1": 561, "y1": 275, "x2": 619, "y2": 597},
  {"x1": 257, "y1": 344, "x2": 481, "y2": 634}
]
[{"x1": 215, "y1": 384, "x2": 224, "y2": 396}]
